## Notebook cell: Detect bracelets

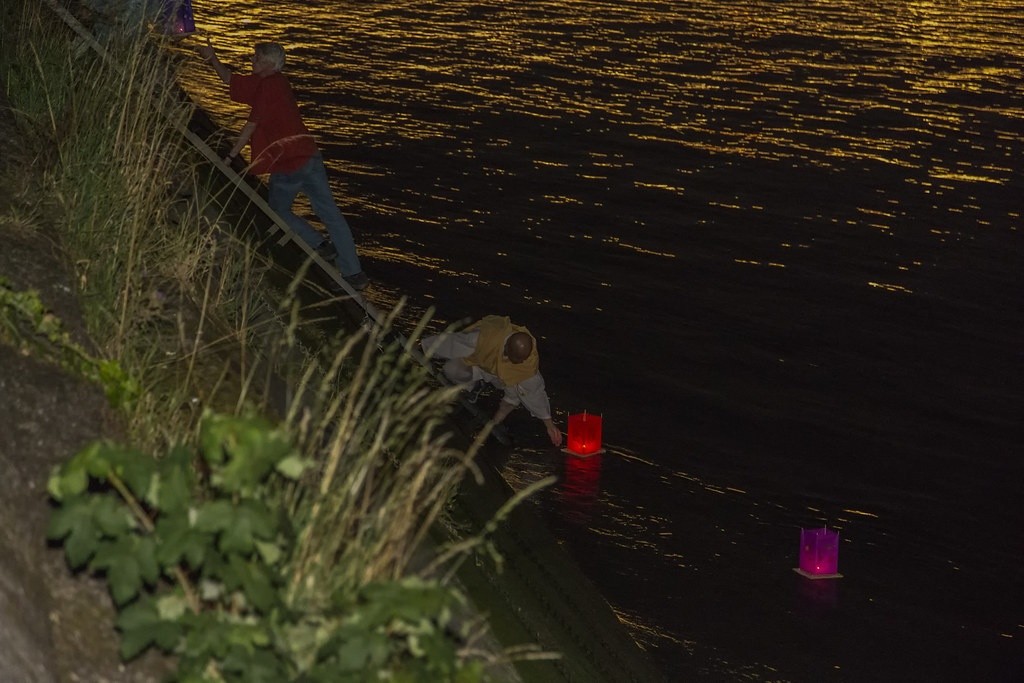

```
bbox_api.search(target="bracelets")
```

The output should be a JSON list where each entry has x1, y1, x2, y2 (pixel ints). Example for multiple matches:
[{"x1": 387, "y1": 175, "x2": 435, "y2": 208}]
[
  {"x1": 418, "y1": 341, "x2": 422, "y2": 351},
  {"x1": 227, "y1": 154, "x2": 235, "y2": 161}
]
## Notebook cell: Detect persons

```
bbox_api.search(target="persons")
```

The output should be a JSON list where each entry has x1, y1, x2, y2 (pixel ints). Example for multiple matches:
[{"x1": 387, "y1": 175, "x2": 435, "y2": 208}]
[
  {"x1": 413, "y1": 315, "x2": 562, "y2": 447},
  {"x1": 196, "y1": 37, "x2": 369, "y2": 290},
  {"x1": 82, "y1": 0, "x2": 186, "y2": 69}
]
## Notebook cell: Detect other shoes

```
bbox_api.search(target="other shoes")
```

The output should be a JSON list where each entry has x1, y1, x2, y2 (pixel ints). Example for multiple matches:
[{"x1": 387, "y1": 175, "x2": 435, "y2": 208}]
[
  {"x1": 331, "y1": 271, "x2": 368, "y2": 294},
  {"x1": 313, "y1": 243, "x2": 337, "y2": 260}
]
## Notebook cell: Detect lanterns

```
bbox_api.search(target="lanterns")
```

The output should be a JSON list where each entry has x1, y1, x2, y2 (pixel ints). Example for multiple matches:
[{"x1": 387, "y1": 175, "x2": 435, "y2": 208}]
[
  {"x1": 160, "y1": 0, "x2": 195, "y2": 40},
  {"x1": 567, "y1": 409, "x2": 602, "y2": 455},
  {"x1": 800, "y1": 525, "x2": 839, "y2": 574}
]
